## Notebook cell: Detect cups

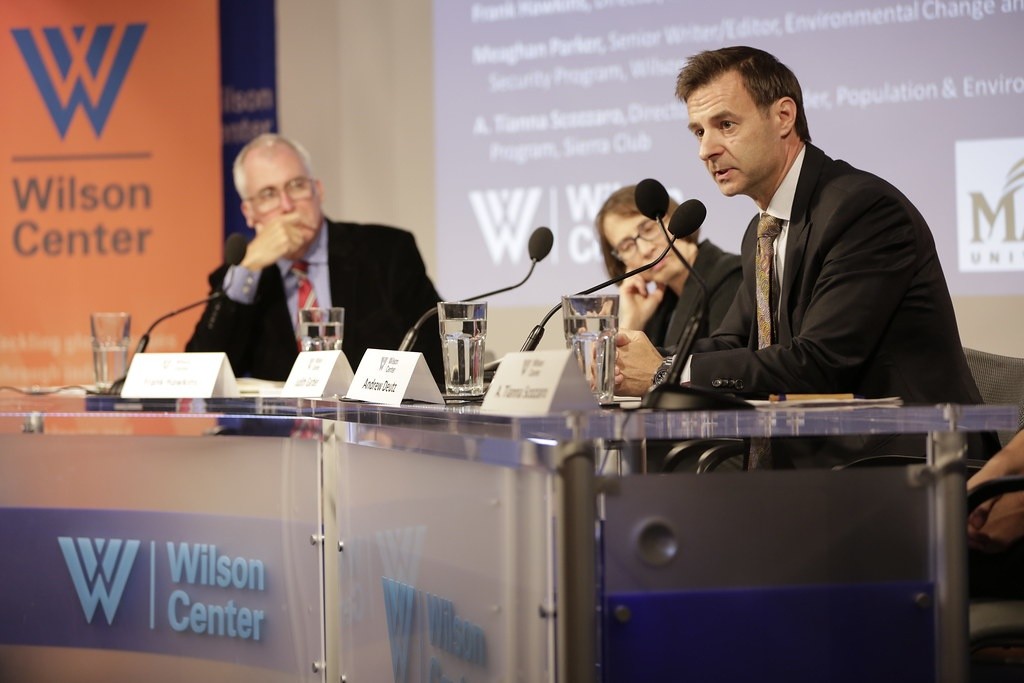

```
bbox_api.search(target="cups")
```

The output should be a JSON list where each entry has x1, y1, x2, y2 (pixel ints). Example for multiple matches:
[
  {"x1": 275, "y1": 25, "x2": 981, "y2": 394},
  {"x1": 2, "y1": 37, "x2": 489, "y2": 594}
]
[
  {"x1": 298, "y1": 306, "x2": 345, "y2": 351},
  {"x1": 90, "y1": 311, "x2": 130, "y2": 390},
  {"x1": 438, "y1": 300, "x2": 488, "y2": 397},
  {"x1": 562, "y1": 294, "x2": 619, "y2": 405}
]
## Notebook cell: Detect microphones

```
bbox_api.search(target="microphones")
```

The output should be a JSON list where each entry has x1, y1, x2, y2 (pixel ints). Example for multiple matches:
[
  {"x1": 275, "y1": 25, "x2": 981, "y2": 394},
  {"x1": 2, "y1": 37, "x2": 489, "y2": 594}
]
[
  {"x1": 519, "y1": 198, "x2": 707, "y2": 353},
  {"x1": 397, "y1": 226, "x2": 554, "y2": 352},
  {"x1": 104, "y1": 233, "x2": 248, "y2": 398},
  {"x1": 626, "y1": 178, "x2": 757, "y2": 411}
]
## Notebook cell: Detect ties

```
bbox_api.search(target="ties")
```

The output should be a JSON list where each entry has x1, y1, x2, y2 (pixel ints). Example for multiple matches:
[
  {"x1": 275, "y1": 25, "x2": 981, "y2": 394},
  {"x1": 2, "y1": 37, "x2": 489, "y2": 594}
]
[
  {"x1": 753, "y1": 214, "x2": 780, "y2": 350},
  {"x1": 290, "y1": 260, "x2": 320, "y2": 351}
]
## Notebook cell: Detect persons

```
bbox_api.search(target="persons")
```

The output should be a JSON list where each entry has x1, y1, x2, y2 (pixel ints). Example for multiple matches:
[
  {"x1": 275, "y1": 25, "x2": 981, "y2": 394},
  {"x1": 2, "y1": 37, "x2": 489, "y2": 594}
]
[
  {"x1": 963, "y1": 397, "x2": 1023, "y2": 604},
  {"x1": 587, "y1": 45, "x2": 1004, "y2": 477},
  {"x1": 591, "y1": 184, "x2": 743, "y2": 349},
  {"x1": 183, "y1": 131, "x2": 448, "y2": 386}
]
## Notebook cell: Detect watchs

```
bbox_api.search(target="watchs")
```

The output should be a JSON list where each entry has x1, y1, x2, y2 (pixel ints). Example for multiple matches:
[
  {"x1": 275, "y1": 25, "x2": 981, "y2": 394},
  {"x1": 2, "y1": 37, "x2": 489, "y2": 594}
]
[{"x1": 650, "y1": 355, "x2": 676, "y2": 387}]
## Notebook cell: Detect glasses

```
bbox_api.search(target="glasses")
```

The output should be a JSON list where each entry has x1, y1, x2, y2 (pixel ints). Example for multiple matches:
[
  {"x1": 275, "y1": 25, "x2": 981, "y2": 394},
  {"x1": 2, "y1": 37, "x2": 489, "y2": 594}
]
[
  {"x1": 609, "y1": 211, "x2": 667, "y2": 260},
  {"x1": 242, "y1": 176, "x2": 314, "y2": 214}
]
